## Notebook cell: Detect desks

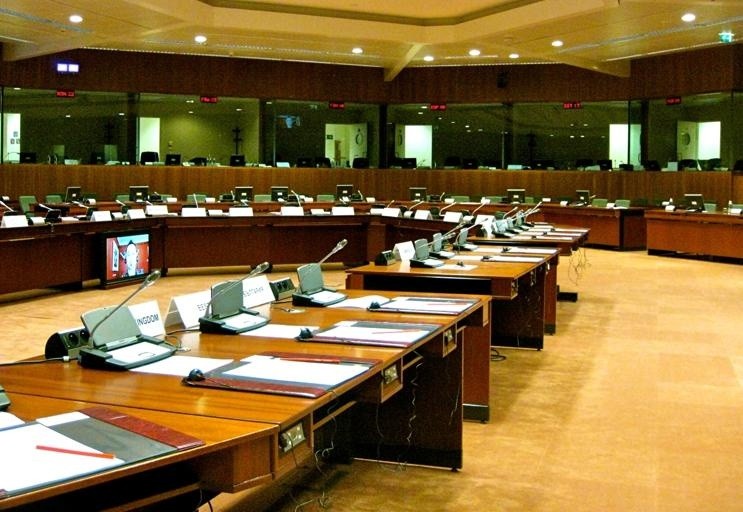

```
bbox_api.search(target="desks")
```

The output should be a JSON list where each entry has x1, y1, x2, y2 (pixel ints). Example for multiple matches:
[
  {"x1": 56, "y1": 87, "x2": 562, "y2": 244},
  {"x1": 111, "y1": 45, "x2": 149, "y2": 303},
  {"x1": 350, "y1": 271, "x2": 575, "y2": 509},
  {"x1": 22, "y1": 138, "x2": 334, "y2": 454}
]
[
  {"x1": 638, "y1": 209, "x2": 742, "y2": 260},
  {"x1": 0, "y1": 189, "x2": 645, "y2": 512}
]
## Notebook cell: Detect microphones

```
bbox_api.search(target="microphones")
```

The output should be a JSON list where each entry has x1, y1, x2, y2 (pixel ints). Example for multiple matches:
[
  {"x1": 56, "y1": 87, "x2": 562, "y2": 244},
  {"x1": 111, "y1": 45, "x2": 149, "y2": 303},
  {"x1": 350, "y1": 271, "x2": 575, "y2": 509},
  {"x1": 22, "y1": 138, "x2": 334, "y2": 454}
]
[
  {"x1": 502, "y1": 200, "x2": 541, "y2": 223},
  {"x1": 72, "y1": 201, "x2": 98, "y2": 220},
  {"x1": 199, "y1": 261, "x2": 271, "y2": 335},
  {"x1": 408, "y1": 200, "x2": 425, "y2": 210},
  {"x1": 194, "y1": 193, "x2": 201, "y2": 209},
  {"x1": 39, "y1": 203, "x2": 62, "y2": 222},
  {"x1": 0, "y1": 200, "x2": 17, "y2": 215},
  {"x1": 116, "y1": 199, "x2": 131, "y2": 217},
  {"x1": 471, "y1": 202, "x2": 488, "y2": 215},
  {"x1": 428, "y1": 221, "x2": 467, "y2": 259},
  {"x1": 292, "y1": 239, "x2": 349, "y2": 307},
  {"x1": 409, "y1": 233, "x2": 456, "y2": 268},
  {"x1": 383, "y1": 199, "x2": 396, "y2": 208},
  {"x1": 439, "y1": 202, "x2": 457, "y2": 217},
  {"x1": 145, "y1": 200, "x2": 154, "y2": 217},
  {"x1": 291, "y1": 190, "x2": 302, "y2": 207},
  {"x1": 77, "y1": 270, "x2": 177, "y2": 371},
  {"x1": 452, "y1": 217, "x2": 489, "y2": 251}
]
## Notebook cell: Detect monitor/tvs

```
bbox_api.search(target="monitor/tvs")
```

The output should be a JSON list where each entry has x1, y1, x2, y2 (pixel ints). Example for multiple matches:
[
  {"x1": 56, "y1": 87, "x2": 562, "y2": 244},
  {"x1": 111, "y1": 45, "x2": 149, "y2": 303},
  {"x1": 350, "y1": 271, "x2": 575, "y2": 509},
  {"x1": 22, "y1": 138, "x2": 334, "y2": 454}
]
[
  {"x1": 409, "y1": 186, "x2": 429, "y2": 201},
  {"x1": 164, "y1": 154, "x2": 181, "y2": 166},
  {"x1": 507, "y1": 189, "x2": 527, "y2": 203},
  {"x1": 574, "y1": 189, "x2": 590, "y2": 204},
  {"x1": 336, "y1": 184, "x2": 354, "y2": 198},
  {"x1": 401, "y1": 158, "x2": 417, "y2": 170},
  {"x1": 269, "y1": 186, "x2": 288, "y2": 201},
  {"x1": 230, "y1": 155, "x2": 246, "y2": 167},
  {"x1": 234, "y1": 186, "x2": 254, "y2": 201},
  {"x1": 64, "y1": 187, "x2": 82, "y2": 202},
  {"x1": 597, "y1": 160, "x2": 613, "y2": 170},
  {"x1": 684, "y1": 194, "x2": 705, "y2": 212},
  {"x1": 463, "y1": 158, "x2": 478, "y2": 169},
  {"x1": 533, "y1": 159, "x2": 554, "y2": 170},
  {"x1": 128, "y1": 185, "x2": 150, "y2": 200}
]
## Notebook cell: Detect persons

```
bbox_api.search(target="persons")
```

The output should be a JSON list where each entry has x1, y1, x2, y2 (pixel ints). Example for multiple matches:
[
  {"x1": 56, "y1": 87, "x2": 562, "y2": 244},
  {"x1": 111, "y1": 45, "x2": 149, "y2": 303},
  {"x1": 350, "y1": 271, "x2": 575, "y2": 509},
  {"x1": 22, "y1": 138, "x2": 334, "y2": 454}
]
[{"x1": 122, "y1": 239, "x2": 138, "y2": 277}]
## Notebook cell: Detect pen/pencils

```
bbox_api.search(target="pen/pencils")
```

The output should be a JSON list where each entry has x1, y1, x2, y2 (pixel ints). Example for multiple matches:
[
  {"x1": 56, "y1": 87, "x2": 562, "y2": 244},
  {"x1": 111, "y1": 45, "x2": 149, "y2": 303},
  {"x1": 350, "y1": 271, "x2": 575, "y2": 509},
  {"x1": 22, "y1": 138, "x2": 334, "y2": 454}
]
[
  {"x1": 427, "y1": 303, "x2": 467, "y2": 305},
  {"x1": 279, "y1": 358, "x2": 341, "y2": 363},
  {"x1": 203, "y1": 376, "x2": 233, "y2": 389},
  {"x1": 371, "y1": 329, "x2": 420, "y2": 334},
  {"x1": 281, "y1": 306, "x2": 290, "y2": 312},
  {"x1": 35, "y1": 445, "x2": 115, "y2": 458}
]
